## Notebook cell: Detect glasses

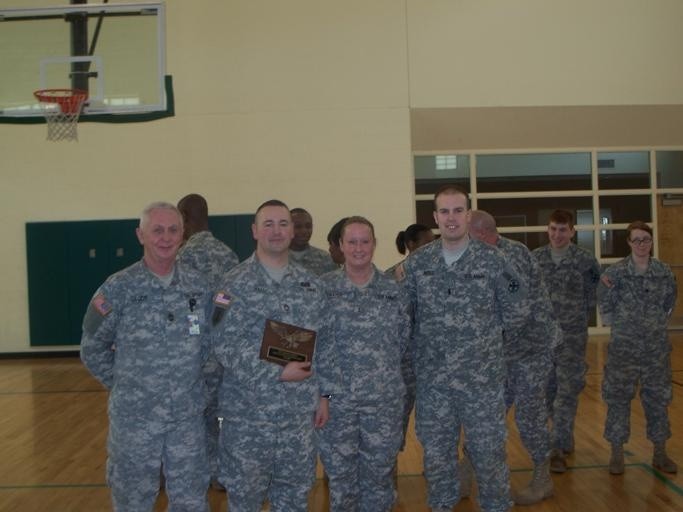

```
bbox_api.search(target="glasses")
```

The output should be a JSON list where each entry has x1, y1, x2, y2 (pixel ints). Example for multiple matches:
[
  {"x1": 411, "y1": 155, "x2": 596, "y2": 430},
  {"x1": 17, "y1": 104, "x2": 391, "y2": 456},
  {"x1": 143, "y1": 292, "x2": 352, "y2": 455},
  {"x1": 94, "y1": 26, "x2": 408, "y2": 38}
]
[{"x1": 628, "y1": 237, "x2": 652, "y2": 245}]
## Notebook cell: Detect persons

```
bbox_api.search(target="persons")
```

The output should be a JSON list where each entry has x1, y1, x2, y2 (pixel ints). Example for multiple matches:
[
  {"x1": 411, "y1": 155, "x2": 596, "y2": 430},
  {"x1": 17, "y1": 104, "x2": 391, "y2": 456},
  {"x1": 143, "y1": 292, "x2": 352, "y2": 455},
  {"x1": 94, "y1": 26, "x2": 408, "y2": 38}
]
[
  {"x1": 316, "y1": 216, "x2": 412, "y2": 509},
  {"x1": 176, "y1": 192, "x2": 243, "y2": 492},
  {"x1": 530, "y1": 208, "x2": 603, "y2": 473},
  {"x1": 79, "y1": 200, "x2": 216, "y2": 510},
  {"x1": 403, "y1": 179, "x2": 533, "y2": 510},
  {"x1": 322, "y1": 220, "x2": 349, "y2": 263},
  {"x1": 205, "y1": 199, "x2": 335, "y2": 512},
  {"x1": 382, "y1": 223, "x2": 436, "y2": 452},
  {"x1": 598, "y1": 221, "x2": 680, "y2": 476},
  {"x1": 468, "y1": 208, "x2": 568, "y2": 506},
  {"x1": 288, "y1": 204, "x2": 339, "y2": 278}
]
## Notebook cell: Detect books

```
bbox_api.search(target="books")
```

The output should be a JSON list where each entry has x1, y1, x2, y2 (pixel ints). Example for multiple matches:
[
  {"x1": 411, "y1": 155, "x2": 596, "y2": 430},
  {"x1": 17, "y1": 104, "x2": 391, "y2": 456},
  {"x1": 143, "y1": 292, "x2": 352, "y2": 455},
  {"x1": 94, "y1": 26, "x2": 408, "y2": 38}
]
[{"x1": 259, "y1": 317, "x2": 317, "y2": 373}]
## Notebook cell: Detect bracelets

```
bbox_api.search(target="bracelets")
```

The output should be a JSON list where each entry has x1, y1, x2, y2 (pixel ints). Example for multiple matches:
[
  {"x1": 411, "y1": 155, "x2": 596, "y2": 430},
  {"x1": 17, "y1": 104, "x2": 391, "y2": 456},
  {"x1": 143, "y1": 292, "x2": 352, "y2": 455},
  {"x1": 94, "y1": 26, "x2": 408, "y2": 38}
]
[{"x1": 320, "y1": 392, "x2": 332, "y2": 401}]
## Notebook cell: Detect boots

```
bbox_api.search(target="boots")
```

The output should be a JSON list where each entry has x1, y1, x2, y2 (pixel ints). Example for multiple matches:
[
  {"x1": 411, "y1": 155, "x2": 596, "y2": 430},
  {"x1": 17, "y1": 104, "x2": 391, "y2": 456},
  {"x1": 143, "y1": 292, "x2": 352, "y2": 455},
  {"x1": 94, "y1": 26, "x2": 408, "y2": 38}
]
[
  {"x1": 457, "y1": 457, "x2": 473, "y2": 496},
  {"x1": 515, "y1": 464, "x2": 553, "y2": 506},
  {"x1": 653, "y1": 441, "x2": 677, "y2": 473},
  {"x1": 610, "y1": 442, "x2": 624, "y2": 474},
  {"x1": 549, "y1": 448, "x2": 566, "y2": 472}
]
